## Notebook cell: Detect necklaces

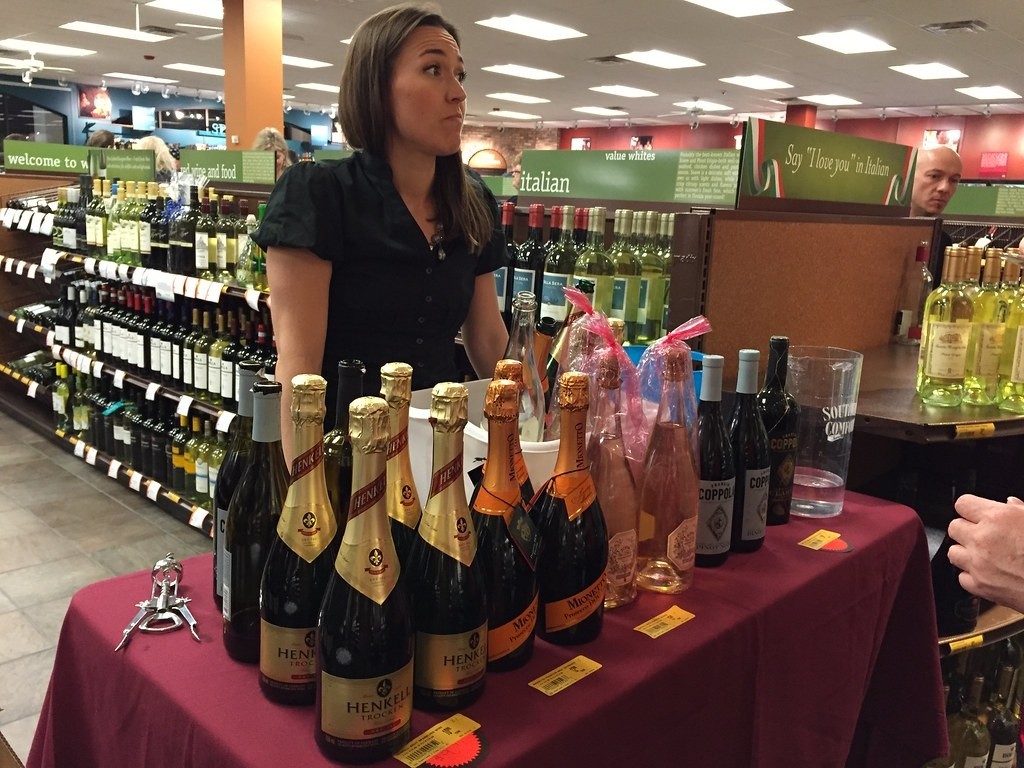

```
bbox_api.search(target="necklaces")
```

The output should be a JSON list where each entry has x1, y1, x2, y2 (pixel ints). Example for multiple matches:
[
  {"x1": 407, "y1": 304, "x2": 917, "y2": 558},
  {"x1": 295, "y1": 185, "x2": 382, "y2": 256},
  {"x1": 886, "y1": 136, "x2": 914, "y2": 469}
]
[{"x1": 431, "y1": 221, "x2": 444, "y2": 244}]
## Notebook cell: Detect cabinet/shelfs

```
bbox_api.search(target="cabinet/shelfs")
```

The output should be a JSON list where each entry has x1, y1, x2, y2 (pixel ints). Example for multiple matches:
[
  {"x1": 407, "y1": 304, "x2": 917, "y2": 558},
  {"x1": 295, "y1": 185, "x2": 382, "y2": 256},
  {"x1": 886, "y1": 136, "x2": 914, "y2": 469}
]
[
  {"x1": 0, "y1": 169, "x2": 272, "y2": 538},
  {"x1": 799, "y1": 342, "x2": 1024, "y2": 659}
]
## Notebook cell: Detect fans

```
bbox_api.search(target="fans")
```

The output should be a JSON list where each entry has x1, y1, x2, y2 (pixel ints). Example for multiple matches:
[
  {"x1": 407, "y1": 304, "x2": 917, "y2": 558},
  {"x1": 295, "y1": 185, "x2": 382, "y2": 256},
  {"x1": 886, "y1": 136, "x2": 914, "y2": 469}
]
[{"x1": 0, "y1": 49, "x2": 75, "y2": 73}]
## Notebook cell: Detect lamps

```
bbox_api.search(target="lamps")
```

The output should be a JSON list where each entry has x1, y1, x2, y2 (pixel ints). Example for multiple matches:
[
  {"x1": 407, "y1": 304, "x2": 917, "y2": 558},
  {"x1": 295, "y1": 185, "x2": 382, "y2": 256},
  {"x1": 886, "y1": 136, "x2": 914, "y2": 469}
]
[{"x1": 21, "y1": 70, "x2": 994, "y2": 130}]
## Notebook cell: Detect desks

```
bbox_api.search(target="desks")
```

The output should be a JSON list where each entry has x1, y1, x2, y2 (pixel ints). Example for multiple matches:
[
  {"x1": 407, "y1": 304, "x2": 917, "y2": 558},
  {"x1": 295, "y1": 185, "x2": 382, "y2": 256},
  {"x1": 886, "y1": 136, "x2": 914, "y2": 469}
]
[{"x1": 26, "y1": 490, "x2": 951, "y2": 768}]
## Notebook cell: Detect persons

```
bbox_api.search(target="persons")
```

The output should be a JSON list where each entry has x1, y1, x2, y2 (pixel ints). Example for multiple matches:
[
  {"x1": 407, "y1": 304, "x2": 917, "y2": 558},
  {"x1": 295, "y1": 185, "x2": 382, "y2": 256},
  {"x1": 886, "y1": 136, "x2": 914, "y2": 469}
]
[
  {"x1": 909, "y1": 144, "x2": 962, "y2": 291},
  {"x1": 134, "y1": 136, "x2": 178, "y2": 184},
  {"x1": 252, "y1": 127, "x2": 293, "y2": 181},
  {"x1": 247, "y1": 3, "x2": 511, "y2": 475},
  {"x1": 948, "y1": 493, "x2": 1024, "y2": 614},
  {"x1": 634, "y1": 141, "x2": 643, "y2": 150},
  {"x1": 506, "y1": 152, "x2": 522, "y2": 206},
  {"x1": 87, "y1": 130, "x2": 115, "y2": 149},
  {"x1": 644, "y1": 141, "x2": 651, "y2": 150}
]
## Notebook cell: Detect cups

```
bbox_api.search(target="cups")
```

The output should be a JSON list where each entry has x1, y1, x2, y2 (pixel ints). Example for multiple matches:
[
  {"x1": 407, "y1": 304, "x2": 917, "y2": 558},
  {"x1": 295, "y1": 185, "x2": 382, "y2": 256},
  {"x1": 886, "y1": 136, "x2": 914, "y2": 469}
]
[{"x1": 784, "y1": 345, "x2": 865, "y2": 519}]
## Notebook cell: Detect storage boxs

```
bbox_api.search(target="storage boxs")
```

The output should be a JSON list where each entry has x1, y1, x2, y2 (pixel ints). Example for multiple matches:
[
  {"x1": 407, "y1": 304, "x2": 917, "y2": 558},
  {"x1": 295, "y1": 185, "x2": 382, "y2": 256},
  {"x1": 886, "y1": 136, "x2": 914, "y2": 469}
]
[{"x1": 408, "y1": 377, "x2": 671, "y2": 509}]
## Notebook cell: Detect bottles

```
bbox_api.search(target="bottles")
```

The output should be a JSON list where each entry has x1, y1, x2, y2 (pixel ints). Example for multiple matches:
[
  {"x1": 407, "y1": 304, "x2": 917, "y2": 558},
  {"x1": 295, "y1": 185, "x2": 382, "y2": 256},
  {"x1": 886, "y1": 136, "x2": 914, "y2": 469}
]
[
  {"x1": 902, "y1": 440, "x2": 1024, "y2": 768},
  {"x1": 891, "y1": 241, "x2": 1024, "y2": 413},
  {"x1": 213, "y1": 280, "x2": 801, "y2": 763},
  {"x1": 5, "y1": 173, "x2": 278, "y2": 516},
  {"x1": 453, "y1": 201, "x2": 676, "y2": 378}
]
